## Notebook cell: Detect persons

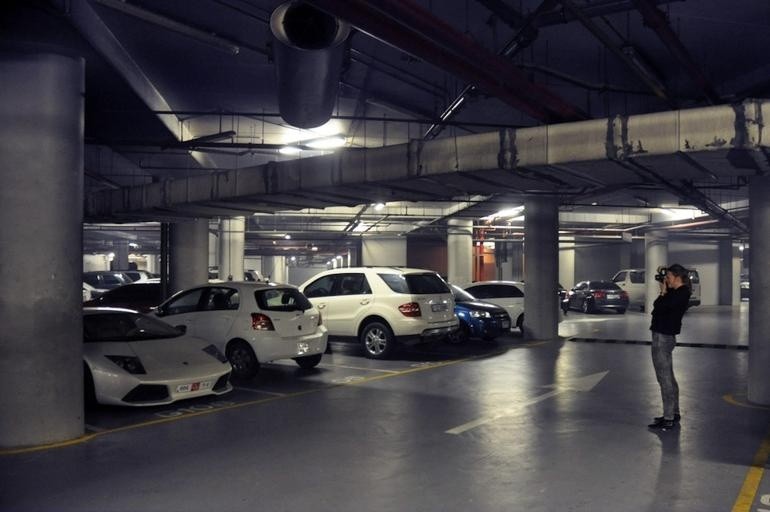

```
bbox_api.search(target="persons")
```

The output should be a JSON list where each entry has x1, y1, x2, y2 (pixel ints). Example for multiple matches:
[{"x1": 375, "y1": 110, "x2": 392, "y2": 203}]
[{"x1": 647, "y1": 263, "x2": 692, "y2": 431}]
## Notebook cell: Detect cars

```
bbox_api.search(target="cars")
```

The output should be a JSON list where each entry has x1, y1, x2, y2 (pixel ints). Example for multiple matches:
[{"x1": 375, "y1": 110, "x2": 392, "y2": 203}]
[{"x1": 739, "y1": 274, "x2": 753, "y2": 301}]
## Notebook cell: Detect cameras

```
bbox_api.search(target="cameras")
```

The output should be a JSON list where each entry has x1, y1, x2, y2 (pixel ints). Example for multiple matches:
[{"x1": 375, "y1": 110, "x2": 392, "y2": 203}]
[{"x1": 655, "y1": 268, "x2": 666, "y2": 283}]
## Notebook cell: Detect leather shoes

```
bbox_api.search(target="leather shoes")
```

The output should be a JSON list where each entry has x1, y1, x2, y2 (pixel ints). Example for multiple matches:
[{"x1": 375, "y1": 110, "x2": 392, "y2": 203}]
[
  {"x1": 655, "y1": 415, "x2": 680, "y2": 422},
  {"x1": 648, "y1": 420, "x2": 674, "y2": 429}
]
